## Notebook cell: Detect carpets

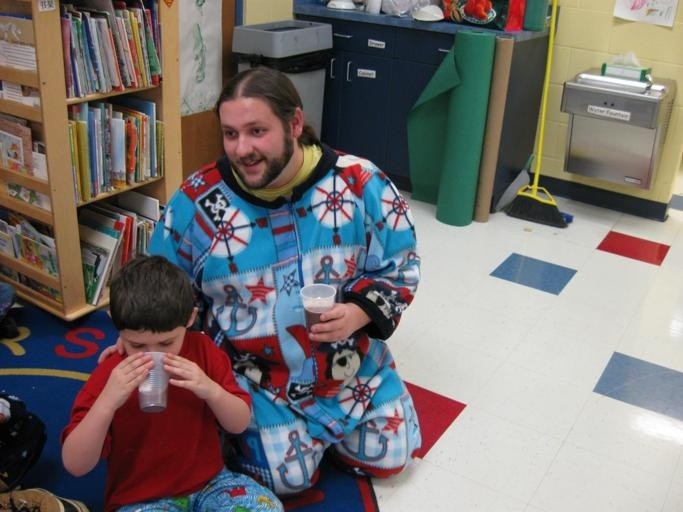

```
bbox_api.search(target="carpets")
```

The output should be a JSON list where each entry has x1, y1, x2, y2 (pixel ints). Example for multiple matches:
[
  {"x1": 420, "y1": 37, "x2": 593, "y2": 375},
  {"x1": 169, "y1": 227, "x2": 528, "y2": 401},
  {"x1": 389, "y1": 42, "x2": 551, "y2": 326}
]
[{"x1": 1, "y1": 305, "x2": 378, "y2": 511}]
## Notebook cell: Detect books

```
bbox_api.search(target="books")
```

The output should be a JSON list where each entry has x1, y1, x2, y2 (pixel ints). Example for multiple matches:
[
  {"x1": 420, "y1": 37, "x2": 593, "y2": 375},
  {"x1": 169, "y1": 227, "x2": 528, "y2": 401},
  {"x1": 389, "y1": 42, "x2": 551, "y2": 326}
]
[
  {"x1": 59, "y1": 1, "x2": 166, "y2": 305},
  {"x1": 1, "y1": 15, "x2": 60, "y2": 302}
]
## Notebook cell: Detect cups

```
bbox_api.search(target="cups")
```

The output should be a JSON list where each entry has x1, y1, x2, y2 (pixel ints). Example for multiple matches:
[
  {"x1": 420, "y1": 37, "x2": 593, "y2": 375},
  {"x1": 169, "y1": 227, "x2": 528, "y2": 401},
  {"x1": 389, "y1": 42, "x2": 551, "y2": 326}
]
[{"x1": 139, "y1": 351, "x2": 168, "y2": 413}]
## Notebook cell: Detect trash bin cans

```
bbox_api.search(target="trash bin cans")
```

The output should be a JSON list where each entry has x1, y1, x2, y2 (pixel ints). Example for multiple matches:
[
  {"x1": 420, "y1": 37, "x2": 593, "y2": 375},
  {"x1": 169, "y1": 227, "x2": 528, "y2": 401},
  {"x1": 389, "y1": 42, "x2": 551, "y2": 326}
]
[{"x1": 230, "y1": 19, "x2": 333, "y2": 142}]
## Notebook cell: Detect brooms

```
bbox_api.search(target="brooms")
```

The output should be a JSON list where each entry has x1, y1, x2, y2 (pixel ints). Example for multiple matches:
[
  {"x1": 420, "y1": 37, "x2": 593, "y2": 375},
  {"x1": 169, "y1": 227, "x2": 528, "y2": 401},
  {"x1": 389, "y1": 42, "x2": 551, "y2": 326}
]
[{"x1": 500, "y1": 0, "x2": 568, "y2": 229}]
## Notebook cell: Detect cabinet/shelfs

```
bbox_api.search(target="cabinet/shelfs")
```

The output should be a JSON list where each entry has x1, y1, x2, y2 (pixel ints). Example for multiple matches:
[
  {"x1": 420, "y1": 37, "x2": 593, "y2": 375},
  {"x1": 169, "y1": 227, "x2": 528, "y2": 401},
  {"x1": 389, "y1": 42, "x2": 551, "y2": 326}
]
[
  {"x1": 0, "y1": 2, "x2": 180, "y2": 321},
  {"x1": 292, "y1": 2, "x2": 557, "y2": 211}
]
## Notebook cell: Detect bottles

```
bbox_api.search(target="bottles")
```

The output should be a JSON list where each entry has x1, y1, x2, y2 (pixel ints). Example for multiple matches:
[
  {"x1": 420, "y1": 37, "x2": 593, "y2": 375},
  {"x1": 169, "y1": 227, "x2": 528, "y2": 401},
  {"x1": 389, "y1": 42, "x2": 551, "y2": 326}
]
[{"x1": 300, "y1": 284, "x2": 336, "y2": 332}]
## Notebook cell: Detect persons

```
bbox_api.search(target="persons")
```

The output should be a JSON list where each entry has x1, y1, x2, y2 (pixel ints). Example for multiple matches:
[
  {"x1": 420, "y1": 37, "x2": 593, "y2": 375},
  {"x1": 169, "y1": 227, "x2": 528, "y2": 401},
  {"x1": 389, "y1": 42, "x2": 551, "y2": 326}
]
[
  {"x1": 60, "y1": 255, "x2": 285, "y2": 512},
  {"x1": 0, "y1": 280, "x2": 19, "y2": 339},
  {"x1": 147, "y1": 67, "x2": 422, "y2": 497}
]
